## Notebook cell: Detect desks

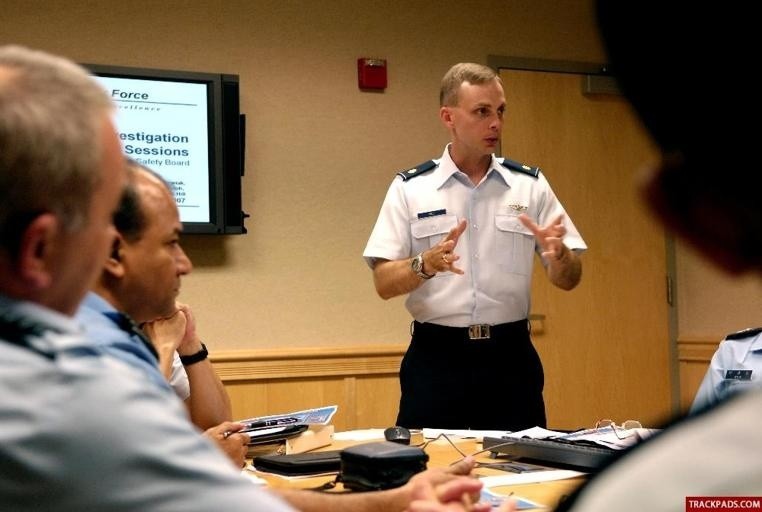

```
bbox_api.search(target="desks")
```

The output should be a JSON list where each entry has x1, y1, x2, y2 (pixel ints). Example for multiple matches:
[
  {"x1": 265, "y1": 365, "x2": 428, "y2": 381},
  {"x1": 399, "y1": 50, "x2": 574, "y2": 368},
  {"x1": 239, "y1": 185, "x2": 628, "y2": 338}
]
[{"x1": 243, "y1": 425, "x2": 590, "y2": 512}]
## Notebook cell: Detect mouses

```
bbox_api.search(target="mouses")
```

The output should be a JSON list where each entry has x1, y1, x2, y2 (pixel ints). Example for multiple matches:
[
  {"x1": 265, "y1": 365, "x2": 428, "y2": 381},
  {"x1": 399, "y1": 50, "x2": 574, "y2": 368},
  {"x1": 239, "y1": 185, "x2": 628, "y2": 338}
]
[{"x1": 384, "y1": 425, "x2": 411, "y2": 444}]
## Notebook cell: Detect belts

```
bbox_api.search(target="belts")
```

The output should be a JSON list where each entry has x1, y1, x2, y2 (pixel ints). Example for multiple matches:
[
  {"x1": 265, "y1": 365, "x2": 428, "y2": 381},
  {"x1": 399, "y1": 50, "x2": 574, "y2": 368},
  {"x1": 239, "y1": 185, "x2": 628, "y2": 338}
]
[{"x1": 414, "y1": 318, "x2": 528, "y2": 340}]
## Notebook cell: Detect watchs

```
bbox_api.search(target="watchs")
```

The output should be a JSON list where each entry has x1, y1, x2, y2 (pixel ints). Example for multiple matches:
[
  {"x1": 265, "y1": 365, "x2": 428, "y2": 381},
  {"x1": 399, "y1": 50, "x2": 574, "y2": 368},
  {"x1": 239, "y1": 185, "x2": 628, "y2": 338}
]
[{"x1": 411, "y1": 252, "x2": 436, "y2": 279}]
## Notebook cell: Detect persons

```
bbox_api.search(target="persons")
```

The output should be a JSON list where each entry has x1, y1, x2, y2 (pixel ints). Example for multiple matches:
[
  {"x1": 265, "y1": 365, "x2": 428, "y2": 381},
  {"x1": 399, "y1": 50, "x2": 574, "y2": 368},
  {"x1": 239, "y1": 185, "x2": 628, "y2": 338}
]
[
  {"x1": 685, "y1": 322, "x2": 762, "y2": 409},
  {"x1": 0, "y1": 41, "x2": 497, "y2": 512},
  {"x1": 75, "y1": 157, "x2": 478, "y2": 512},
  {"x1": 362, "y1": 59, "x2": 589, "y2": 432},
  {"x1": 134, "y1": 301, "x2": 233, "y2": 436},
  {"x1": 552, "y1": 2, "x2": 762, "y2": 512}
]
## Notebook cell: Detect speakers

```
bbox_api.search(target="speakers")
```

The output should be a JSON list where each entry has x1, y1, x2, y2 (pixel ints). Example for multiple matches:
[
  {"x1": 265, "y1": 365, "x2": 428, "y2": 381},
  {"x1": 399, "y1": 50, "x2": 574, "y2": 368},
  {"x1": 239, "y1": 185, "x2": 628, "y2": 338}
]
[{"x1": 221, "y1": 73, "x2": 243, "y2": 234}]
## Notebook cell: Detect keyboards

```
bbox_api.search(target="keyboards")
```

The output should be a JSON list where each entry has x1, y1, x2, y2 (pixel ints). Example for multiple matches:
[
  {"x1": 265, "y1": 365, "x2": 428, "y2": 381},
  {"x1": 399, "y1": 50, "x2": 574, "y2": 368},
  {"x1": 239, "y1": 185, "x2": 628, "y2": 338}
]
[{"x1": 482, "y1": 435, "x2": 616, "y2": 470}]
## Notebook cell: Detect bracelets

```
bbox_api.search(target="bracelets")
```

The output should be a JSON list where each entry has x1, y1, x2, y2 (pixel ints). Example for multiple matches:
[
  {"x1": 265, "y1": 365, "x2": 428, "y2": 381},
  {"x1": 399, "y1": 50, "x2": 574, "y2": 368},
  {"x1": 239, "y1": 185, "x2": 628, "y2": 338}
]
[{"x1": 179, "y1": 342, "x2": 209, "y2": 365}]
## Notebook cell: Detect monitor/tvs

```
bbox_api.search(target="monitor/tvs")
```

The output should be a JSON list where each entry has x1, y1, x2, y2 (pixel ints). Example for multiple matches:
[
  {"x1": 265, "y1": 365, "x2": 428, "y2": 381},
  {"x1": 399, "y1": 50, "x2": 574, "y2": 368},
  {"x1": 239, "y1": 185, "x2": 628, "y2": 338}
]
[{"x1": 75, "y1": 61, "x2": 224, "y2": 236}]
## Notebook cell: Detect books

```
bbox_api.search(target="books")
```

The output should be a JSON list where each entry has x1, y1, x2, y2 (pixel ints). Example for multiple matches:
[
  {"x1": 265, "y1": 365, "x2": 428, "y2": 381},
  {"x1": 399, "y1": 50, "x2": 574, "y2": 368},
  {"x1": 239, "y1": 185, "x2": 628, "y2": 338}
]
[
  {"x1": 240, "y1": 422, "x2": 311, "y2": 446},
  {"x1": 506, "y1": 421, "x2": 649, "y2": 452}
]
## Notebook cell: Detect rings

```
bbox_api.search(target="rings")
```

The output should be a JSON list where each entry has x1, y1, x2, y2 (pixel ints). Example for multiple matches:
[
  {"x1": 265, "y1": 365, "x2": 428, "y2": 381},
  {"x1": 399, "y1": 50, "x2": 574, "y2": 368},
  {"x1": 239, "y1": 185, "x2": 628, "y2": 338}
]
[{"x1": 441, "y1": 253, "x2": 449, "y2": 263}]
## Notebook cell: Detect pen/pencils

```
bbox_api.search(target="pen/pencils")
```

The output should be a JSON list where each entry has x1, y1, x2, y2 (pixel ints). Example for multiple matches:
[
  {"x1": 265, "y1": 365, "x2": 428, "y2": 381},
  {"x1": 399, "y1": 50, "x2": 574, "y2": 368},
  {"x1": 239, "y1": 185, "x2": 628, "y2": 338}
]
[
  {"x1": 244, "y1": 416, "x2": 299, "y2": 428},
  {"x1": 221, "y1": 432, "x2": 232, "y2": 438}
]
